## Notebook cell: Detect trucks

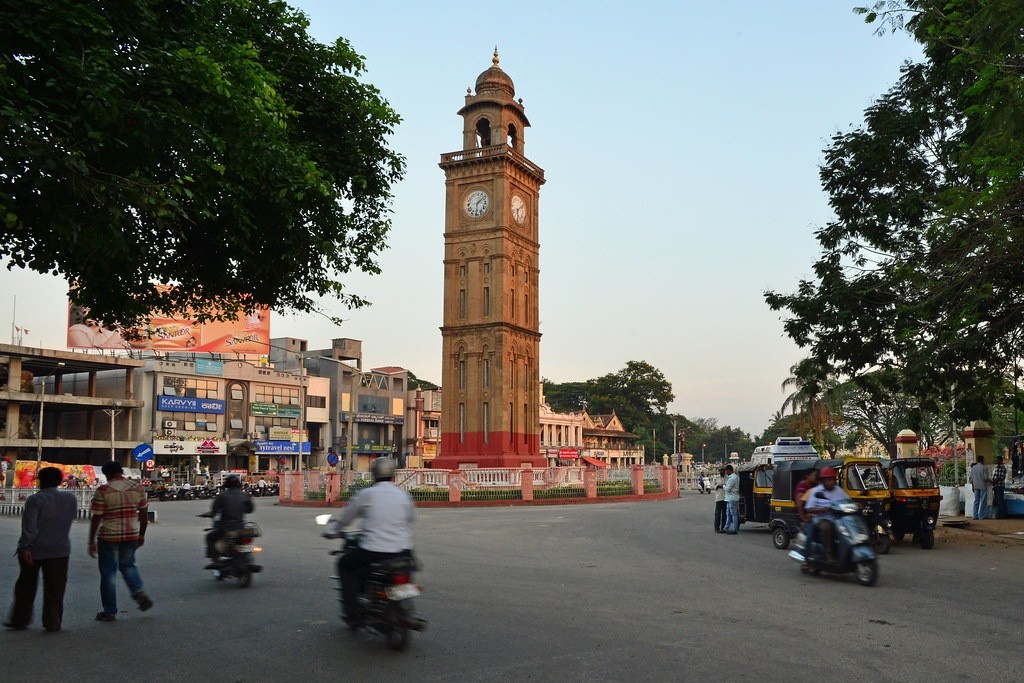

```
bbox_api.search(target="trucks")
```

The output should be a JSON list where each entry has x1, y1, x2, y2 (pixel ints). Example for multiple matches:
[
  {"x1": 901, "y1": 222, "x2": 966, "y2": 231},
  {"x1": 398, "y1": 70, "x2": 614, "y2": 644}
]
[{"x1": 750, "y1": 437, "x2": 819, "y2": 463}]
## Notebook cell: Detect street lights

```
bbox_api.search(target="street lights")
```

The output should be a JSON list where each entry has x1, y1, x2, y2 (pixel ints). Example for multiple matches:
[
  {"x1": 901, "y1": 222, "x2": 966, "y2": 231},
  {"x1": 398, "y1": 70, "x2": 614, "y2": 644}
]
[
  {"x1": 36, "y1": 362, "x2": 65, "y2": 490},
  {"x1": 306, "y1": 355, "x2": 359, "y2": 490},
  {"x1": 702, "y1": 444, "x2": 705, "y2": 462}
]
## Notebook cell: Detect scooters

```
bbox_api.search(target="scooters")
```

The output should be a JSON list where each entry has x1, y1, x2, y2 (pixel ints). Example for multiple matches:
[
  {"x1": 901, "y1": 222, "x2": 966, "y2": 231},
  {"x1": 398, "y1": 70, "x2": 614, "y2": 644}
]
[
  {"x1": 697, "y1": 477, "x2": 712, "y2": 495},
  {"x1": 790, "y1": 491, "x2": 878, "y2": 586}
]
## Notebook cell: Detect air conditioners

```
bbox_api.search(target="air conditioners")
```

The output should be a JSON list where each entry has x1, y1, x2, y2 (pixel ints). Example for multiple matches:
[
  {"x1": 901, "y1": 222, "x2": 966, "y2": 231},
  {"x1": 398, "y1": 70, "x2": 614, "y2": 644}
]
[
  {"x1": 164, "y1": 429, "x2": 176, "y2": 436},
  {"x1": 251, "y1": 432, "x2": 261, "y2": 438},
  {"x1": 163, "y1": 421, "x2": 177, "y2": 429}
]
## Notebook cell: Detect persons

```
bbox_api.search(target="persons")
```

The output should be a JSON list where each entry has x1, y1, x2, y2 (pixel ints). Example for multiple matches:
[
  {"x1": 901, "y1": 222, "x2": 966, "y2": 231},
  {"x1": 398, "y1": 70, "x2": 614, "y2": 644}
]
[
  {"x1": 161, "y1": 465, "x2": 169, "y2": 478},
  {"x1": 153, "y1": 481, "x2": 161, "y2": 490},
  {"x1": 184, "y1": 482, "x2": 190, "y2": 489},
  {"x1": 257, "y1": 477, "x2": 267, "y2": 497},
  {"x1": 795, "y1": 468, "x2": 850, "y2": 563},
  {"x1": 1, "y1": 467, "x2": 78, "y2": 630},
  {"x1": 699, "y1": 472, "x2": 705, "y2": 490},
  {"x1": 68, "y1": 303, "x2": 128, "y2": 346},
  {"x1": 87, "y1": 460, "x2": 154, "y2": 620},
  {"x1": 204, "y1": 475, "x2": 254, "y2": 570},
  {"x1": 711, "y1": 465, "x2": 740, "y2": 535},
  {"x1": 323, "y1": 457, "x2": 415, "y2": 627},
  {"x1": 67, "y1": 475, "x2": 100, "y2": 488},
  {"x1": 970, "y1": 454, "x2": 991, "y2": 520},
  {"x1": 203, "y1": 476, "x2": 214, "y2": 489},
  {"x1": 276, "y1": 475, "x2": 279, "y2": 483},
  {"x1": 993, "y1": 456, "x2": 1006, "y2": 519}
]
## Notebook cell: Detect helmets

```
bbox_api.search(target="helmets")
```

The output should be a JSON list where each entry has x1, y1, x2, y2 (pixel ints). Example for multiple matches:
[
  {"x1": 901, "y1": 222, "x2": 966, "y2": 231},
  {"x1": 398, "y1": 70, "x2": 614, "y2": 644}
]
[
  {"x1": 373, "y1": 457, "x2": 395, "y2": 478},
  {"x1": 820, "y1": 467, "x2": 835, "y2": 477}
]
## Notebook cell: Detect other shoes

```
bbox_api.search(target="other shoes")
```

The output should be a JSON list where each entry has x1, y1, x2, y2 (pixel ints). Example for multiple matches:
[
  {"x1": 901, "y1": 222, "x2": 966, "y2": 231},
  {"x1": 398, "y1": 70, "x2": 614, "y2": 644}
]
[
  {"x1": 728, "y1": 530, "x2": 737, "y2": 535},
  {"x1": 133, "y1": 590, "x2": 154, "y2": 611},
  {"x1": 204, "y1": 564, "x2": 219, "y2": 570},
  {"x1": 96, "y1": 610, "x2": 115, "y2": 622},
  {"x1": 717, "y1": 530, "x2": 726, "y2": 533},
  {"x1": 3, "y1": 620, "x2": 29, "y2": 630}
]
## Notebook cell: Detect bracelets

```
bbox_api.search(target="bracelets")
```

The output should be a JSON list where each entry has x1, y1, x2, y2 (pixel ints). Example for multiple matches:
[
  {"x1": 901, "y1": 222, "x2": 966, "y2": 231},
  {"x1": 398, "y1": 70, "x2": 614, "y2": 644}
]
[{"x1": 88, "y1": 542, "x2": 94, "y2": 544}]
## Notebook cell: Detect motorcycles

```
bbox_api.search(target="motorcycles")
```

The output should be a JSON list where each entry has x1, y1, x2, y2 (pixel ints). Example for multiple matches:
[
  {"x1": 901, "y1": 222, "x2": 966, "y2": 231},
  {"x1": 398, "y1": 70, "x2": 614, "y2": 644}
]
[
  {"x1": 158, "y1": 485, "x2": 280, "y2": 502},
  {"x1": 196, "y1": 511, "x2": 262, "y2": 586},
  {"x1": 315, "y1": 512, "x2": 427, "y2": 651},
  {"x1": 768, "y1": 456, "x2": 896, "y2": 553},
  {"x1": 875, "y1": 457, "x2": 943, "y2": 549},
  {"x1": 734, "y1": 462, "x2": 774, "y2": 523},
  {"x1": 60, "y1": 479, "x2": 91, "y2": 493}
]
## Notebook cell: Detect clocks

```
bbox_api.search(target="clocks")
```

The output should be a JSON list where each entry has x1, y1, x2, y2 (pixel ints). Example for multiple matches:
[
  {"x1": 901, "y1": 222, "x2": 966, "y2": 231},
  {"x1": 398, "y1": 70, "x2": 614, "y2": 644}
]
[
  {"x1": 464, "y1": 191, "x2": 488, "y2": 217},
  {"x1": 511, "y1": 195, "x2": 526, "y2": 223}
]
